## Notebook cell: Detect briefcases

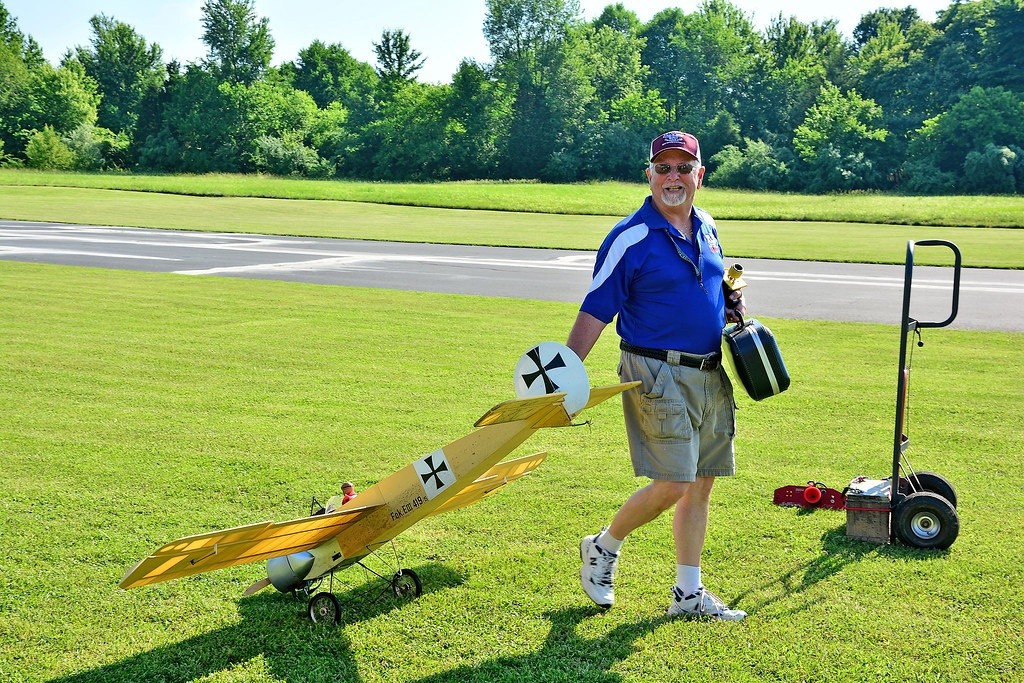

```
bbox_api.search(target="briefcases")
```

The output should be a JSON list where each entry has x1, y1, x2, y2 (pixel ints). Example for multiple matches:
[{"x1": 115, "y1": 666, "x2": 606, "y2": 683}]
[{"x1": 722, "y1": 309, "x2": 790, "y2": 402}]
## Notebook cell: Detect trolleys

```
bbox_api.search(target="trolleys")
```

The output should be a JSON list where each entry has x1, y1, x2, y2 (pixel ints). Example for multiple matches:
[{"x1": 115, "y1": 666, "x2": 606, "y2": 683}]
[{"x1": 888, "y1": 240, "x2": 962, "y2": 550}]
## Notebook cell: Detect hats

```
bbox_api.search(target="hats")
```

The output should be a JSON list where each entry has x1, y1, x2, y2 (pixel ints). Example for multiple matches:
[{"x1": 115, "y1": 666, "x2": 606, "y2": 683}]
[{"x1": 650, "y1": 131, "x2": 701, "y2": 164}]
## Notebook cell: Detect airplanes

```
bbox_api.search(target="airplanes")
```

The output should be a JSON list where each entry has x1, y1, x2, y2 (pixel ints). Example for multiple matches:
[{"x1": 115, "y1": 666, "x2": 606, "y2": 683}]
[{"x1": 120, "y1": 343, "x2": 643, "y2": 627}]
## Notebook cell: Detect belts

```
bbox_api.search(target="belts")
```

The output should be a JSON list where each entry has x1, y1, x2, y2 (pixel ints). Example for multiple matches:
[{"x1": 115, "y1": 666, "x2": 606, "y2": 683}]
[{"x1": 621, "y1": 340, "x2": 722, "y2": 371}]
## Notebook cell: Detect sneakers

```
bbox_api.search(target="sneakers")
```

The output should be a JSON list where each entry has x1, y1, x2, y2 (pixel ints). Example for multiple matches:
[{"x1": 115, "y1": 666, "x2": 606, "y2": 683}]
[
  {"x1": 580, "y1": 526, "x2": 621, "y2": 608},
  {"x1": 668, "y1": 585, "x2": 748, "y2": 622}
]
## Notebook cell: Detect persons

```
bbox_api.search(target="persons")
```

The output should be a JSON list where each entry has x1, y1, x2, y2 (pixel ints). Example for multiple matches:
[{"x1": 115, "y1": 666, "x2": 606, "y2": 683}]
[
  {"x1": 341, "y1": 482, "x2": 357, "y2": 505},
  {"x1": 565, "y1": 131, "x2": 748, "y2": 621}
]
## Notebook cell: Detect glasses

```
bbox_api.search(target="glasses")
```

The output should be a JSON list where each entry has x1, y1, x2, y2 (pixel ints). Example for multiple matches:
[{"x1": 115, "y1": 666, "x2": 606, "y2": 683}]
[{"x1": 655, "y1": 163, "x2": 699, "y2": 174}]
